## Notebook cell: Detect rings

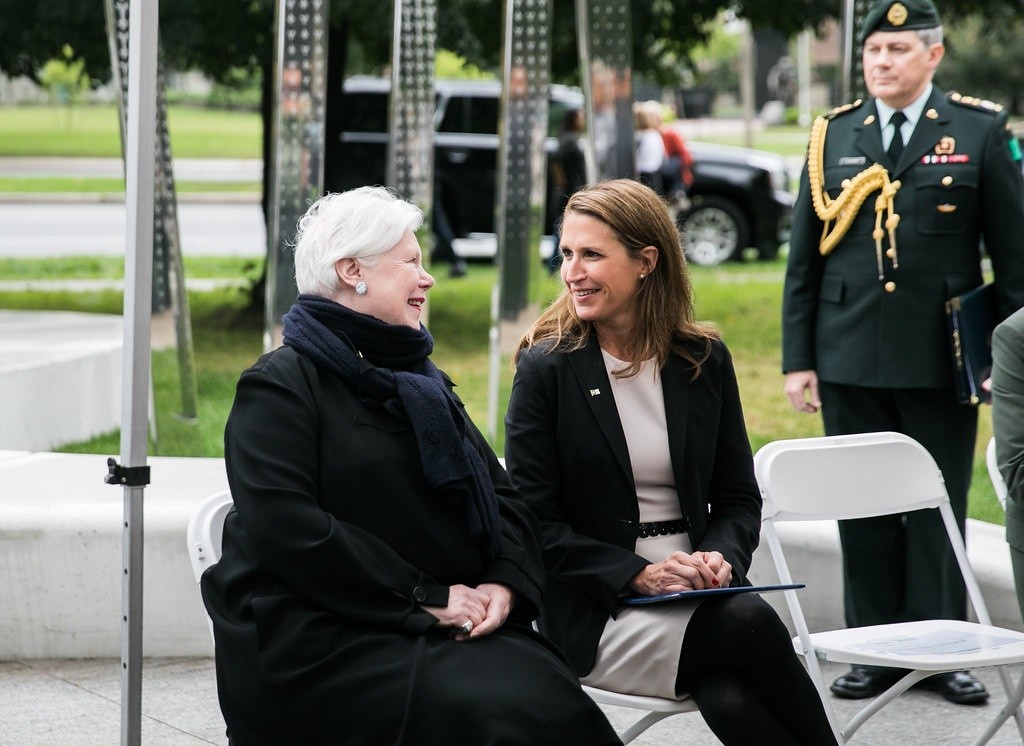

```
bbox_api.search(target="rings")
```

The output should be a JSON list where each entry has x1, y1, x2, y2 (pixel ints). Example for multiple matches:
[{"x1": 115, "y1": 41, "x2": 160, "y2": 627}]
[{"x1": 460, "y1": 620, "x2": 473, "y2": 634}]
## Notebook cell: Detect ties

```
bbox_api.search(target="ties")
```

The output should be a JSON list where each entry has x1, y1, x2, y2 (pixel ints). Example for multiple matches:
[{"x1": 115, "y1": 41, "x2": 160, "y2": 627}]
[{"x1": 887, "y1": 111, "x2": 908, "y2": 162}]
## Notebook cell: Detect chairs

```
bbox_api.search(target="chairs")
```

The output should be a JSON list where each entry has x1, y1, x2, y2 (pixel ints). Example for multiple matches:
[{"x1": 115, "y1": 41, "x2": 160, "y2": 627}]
[{"x1": 186, "y1": 431, "x2": 1024, "y2": 746}]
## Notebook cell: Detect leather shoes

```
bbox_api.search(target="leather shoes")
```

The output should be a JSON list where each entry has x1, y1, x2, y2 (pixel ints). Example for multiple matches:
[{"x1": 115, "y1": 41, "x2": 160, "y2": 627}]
[
  {"x1": 830, "y1": 670, "x2": 884, "y2": 701},
  {"x1": 910, "y1": 671, "x2": 990, "y2": 705}
]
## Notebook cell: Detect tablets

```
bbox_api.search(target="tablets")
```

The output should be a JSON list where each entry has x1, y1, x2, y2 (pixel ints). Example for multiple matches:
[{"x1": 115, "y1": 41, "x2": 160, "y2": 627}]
[{"x1": 628, "y1": 584, "x2": 806, "y2": 605}]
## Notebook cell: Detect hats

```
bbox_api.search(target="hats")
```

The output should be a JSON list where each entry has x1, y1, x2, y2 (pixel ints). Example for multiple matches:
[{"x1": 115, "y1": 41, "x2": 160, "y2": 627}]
[{"x1": 859, "y1": 0, "x2": 940, "y2": 44}]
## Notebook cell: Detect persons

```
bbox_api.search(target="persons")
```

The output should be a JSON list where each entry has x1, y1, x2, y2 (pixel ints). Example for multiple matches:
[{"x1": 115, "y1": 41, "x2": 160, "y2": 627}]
[
  {"x1": 200, "y1": 184, "x2": 624, "y2": 746},
  {"x1": 432, "y1": 170, "x2": 465, "y2": 278},
  {"x1": 632, "y1": 100, "x2": 696, "y2": 206},
  {"x1": 542, "y1": 109, "x2": 587, "y2": 277},
  {"x1": 507, "y1": 177, "x2": 839, "y2": 746},
  {"x1": 783, "y1": 0, "x2": 1024, "y2": 706},
  {"x1": 992, "y1": 306, "x2": 1024, "y2": 626}
]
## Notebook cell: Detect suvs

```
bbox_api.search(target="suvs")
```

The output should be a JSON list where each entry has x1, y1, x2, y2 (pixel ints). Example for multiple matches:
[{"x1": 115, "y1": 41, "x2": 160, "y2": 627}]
[{"x1": 336, "y1": 77, "x2": 797, "y2": 271}]
